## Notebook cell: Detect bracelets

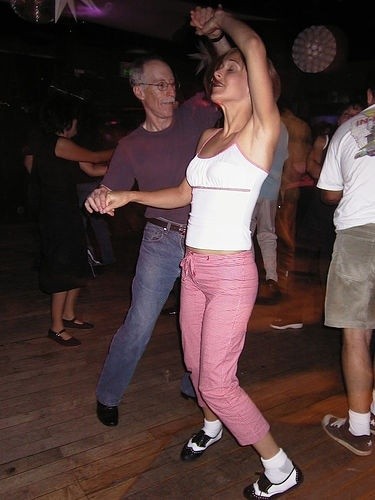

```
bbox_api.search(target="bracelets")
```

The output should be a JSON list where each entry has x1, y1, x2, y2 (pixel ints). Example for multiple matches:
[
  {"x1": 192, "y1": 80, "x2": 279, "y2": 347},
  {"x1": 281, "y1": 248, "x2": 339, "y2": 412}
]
[{"x1": 208, "y1": 31, "x2": 224, "y2": 42}]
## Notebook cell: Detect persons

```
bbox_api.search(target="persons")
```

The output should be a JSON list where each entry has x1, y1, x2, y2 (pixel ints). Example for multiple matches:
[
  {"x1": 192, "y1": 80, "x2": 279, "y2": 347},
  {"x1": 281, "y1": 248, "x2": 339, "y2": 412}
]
[
  {"x1": 0, "y1": 90, "x2": 375, "y2": 347},
  {"x1": 84, "y1": 7, "x2": 233, "y2": 427},
  {"x1": 316, "y1": 89, "x2": 374, "y2": 456},
  {"x1": 99, "y1": 4, "x2": 305, "y2": 499}
]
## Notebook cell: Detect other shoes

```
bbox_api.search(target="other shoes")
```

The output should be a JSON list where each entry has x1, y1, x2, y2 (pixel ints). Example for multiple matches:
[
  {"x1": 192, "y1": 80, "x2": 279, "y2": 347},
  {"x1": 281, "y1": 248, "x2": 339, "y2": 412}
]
[
  {"x1": 96, "y1": 400, "x2": 119, "y2": 426},
  {"x1": 48, "y1": 328, "x2": 80, "y2": 346},
  {"x1": 256, "y1": 280, "x2": 280, "y2": 301},
  {"x1": 270, "y1": 317, "x2": 304, "y2": 330},
  {"x1": 62, "y1": 317, "x2": 94, "y2": 329},
  {"x1": 180, "y1": 390, "x2": 198, "y2": 400}
]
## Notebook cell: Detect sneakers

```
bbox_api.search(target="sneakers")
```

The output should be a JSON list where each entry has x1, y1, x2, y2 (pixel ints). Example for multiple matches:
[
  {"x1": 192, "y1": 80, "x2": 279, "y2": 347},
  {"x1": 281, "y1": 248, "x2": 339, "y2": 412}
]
[
  {"x1": 179, "y1": 427, "x2": 224, "y2": 462},
  {"x1": 243, "y1": 460, "x2": 304, "y2": 500},
  {"x1": 322, "y1": 411, "x2": 375, "y2": 456}
]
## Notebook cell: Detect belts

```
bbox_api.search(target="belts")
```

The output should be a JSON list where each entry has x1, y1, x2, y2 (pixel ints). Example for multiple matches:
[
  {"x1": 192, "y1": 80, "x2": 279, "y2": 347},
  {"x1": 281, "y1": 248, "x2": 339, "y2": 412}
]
[{"x1": 148, "y1": 218, "x2": 187, "y2": 235}]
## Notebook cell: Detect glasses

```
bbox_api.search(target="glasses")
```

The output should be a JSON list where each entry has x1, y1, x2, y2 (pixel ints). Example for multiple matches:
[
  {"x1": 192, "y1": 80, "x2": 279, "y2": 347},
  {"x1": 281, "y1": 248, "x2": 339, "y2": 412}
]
[{"x1": 139, "y1": 81, "x2": 181, "y2": 91}]
